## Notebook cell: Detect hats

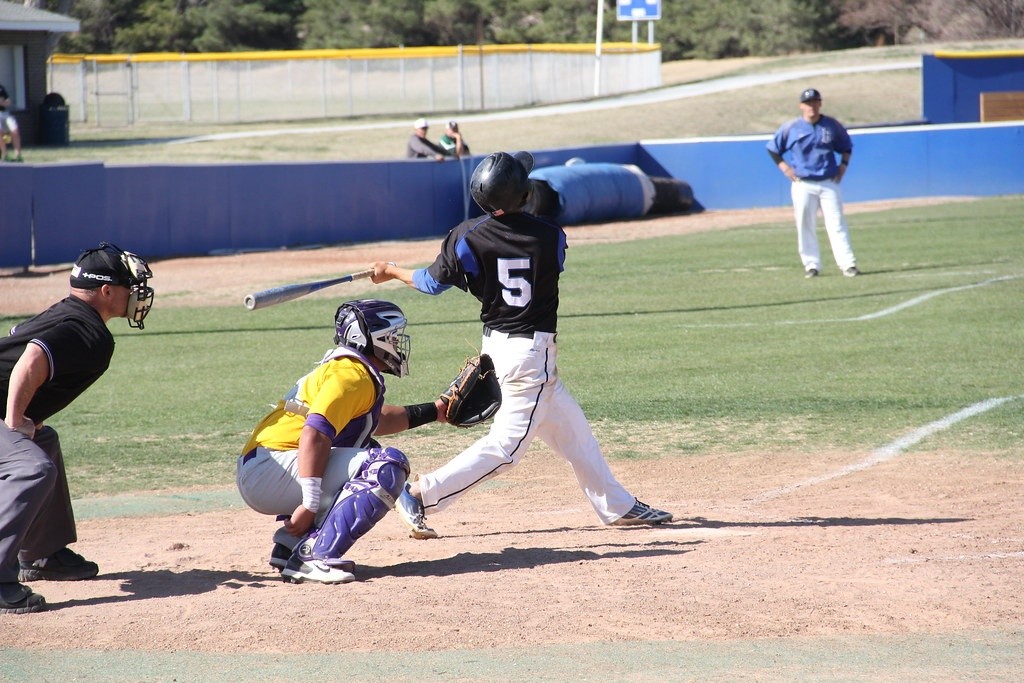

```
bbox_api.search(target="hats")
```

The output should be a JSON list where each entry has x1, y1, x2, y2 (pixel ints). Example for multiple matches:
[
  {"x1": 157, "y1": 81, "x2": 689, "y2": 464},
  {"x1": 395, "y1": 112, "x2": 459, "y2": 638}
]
[
  {"x1": 801, "y1": 89, "x2": 821, "y2": 102},
  {"x1": 70, "y1": 249, "x2": 146, "y2": 289}
]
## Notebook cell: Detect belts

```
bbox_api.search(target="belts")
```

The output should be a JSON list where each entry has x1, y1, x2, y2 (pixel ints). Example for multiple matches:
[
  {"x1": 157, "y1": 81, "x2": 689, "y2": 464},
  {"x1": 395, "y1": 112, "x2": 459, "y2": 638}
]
[{"x1": 482, "y1": 326, "x2": 534, "y2": 339}]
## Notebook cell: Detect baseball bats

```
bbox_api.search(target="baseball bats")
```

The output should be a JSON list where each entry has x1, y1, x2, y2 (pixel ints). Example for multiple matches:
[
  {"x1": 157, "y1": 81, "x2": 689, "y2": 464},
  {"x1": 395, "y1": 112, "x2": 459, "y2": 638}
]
[{"x1": 242, "y1": 260, "x2": 396, "y2": 311}]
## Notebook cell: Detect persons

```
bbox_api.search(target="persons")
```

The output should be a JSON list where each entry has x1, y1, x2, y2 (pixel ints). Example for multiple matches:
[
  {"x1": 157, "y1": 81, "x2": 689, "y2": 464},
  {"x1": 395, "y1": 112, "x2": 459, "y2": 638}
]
[
  {"x1": 237, "y1": 298, "x2": 502, "y2": 585},
  {"x1": 766, "y1": 88, "x2": 859, "y2": 278},
  {"x1": 0, "y1": 243, "x2": 154, "y2": 615},
  {"x1": 405, "y1": 119, "x2": 446, "y2": 161},
  {"x1": 439, "y1": 121, "x2": 470, "y2": 156},
  {"x1": 368, "y1": 150, "x2": 673, "y2": 539},
  {"x1": 0, "y1": 84, "x2": 23, "y2": 160}
]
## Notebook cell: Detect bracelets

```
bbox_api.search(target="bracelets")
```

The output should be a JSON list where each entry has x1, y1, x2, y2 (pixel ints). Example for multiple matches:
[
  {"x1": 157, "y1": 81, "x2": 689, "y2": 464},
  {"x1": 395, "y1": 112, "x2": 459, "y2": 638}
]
[
  {"x1": 300, "y1": 476, "x2": 322, "y2": 514},
  {"x1": 402, "y1": 401, "x2": 438, "y2": 430},
  {"x1": 840, "y1": 159, "x2": 849, "y2": 165}
]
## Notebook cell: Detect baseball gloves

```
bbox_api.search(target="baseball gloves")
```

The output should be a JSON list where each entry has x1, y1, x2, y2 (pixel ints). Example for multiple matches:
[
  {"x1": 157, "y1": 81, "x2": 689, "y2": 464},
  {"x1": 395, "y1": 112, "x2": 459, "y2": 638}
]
[{"x1": 438, "y1": 338, "x2": 503, "y2": 429}]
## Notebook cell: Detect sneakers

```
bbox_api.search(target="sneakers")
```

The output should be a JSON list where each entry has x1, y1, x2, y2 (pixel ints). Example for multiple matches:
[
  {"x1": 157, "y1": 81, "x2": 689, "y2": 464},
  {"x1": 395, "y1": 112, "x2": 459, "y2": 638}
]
[
  {"x1": 843, "y1": 267, "x2": 861, "y2": 275},
  {"x1": 805, "y1": 269, "x2": 817, "y2": 278},
  {"x1": 612, "y1": 499, "x2": 673, "y2": 524},
  {"x1": 269, "y1": 543, "x2": 291, "y2": 566},
  {"x1": 395, "y1": 483, "x2": 437, "y2": 536},
  {"x1": 281, "y1": 554, "x2": 354, "y2": 584},
  {"x1": 0, "y1": 583, "x2": 45, "y2": 614},
  {"x1": 18, "y1": 548, "x2": 99, "y2": 582}
]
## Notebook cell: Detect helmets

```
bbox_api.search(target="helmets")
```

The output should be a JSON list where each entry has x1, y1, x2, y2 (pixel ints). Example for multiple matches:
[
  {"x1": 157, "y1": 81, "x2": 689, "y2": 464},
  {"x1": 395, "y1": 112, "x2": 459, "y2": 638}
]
[
  {"x1": 336, "y1": 299, "x2": 406, "y2": 379},
  {"x1": 470, "y1": 150, "x2": 533, "y2": 216}
]
